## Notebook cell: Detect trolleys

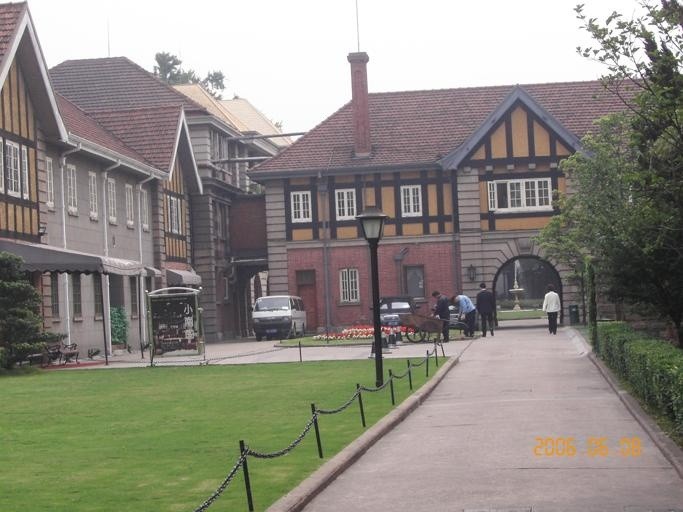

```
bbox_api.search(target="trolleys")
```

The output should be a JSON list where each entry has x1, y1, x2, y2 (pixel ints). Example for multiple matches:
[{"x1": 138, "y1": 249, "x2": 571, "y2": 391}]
[{"x1": 398, "y1": 313, "x2": 469, "y2": 343}]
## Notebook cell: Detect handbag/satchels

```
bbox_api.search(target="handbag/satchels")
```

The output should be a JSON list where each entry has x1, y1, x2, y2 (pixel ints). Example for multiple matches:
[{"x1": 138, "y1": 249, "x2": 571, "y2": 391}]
[{"x1": 494, "y1": 317, "x2": 498, "y2": 327}]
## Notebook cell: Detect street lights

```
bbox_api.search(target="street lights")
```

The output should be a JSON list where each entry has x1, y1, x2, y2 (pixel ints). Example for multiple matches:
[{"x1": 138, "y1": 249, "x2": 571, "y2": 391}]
[{"x1": 355, "y1": 205, "x2": 389, "y2": 389}]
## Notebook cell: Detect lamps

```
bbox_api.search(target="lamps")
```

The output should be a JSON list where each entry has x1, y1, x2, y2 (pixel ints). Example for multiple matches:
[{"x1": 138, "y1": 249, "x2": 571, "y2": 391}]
[{"x1": 467, "y1": 264, "x2": 477, "y2": 282}]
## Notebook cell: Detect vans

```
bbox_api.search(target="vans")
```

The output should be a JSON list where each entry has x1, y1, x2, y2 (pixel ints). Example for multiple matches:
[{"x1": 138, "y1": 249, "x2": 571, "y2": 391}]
[{"x1": 251, "y1": 295, "x2": 307, "y2": 341}]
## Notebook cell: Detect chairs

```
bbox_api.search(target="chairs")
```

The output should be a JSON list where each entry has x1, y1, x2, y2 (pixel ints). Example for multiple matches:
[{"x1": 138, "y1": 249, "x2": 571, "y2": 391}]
[{"x1": 42, "y1": 341, "x2": 80, "y2": 368}]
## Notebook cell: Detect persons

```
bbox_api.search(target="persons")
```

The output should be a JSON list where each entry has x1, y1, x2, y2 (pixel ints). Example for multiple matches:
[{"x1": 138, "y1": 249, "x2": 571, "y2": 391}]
[
  {"x1": 543, "y1": 283, "x2": 561, "y2": 335},
  {"x1": 432, "y1": 290, "x2": 449, "y2": 342},
  {"x1": 451, "y1": 294, "x2": 476, "y2": 337},
  {"x1": 476, "y1": 283, "x2": 496, "y2": 337}
]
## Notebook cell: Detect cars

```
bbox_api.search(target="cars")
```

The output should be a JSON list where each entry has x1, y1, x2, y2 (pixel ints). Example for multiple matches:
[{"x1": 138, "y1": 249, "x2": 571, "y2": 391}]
[
  {"x1": 379, "y1": 296, "x2": 417, "y2": 326},
  {"x1": 431, "y1": 303, "x2": 465, "y2": 329}
]
